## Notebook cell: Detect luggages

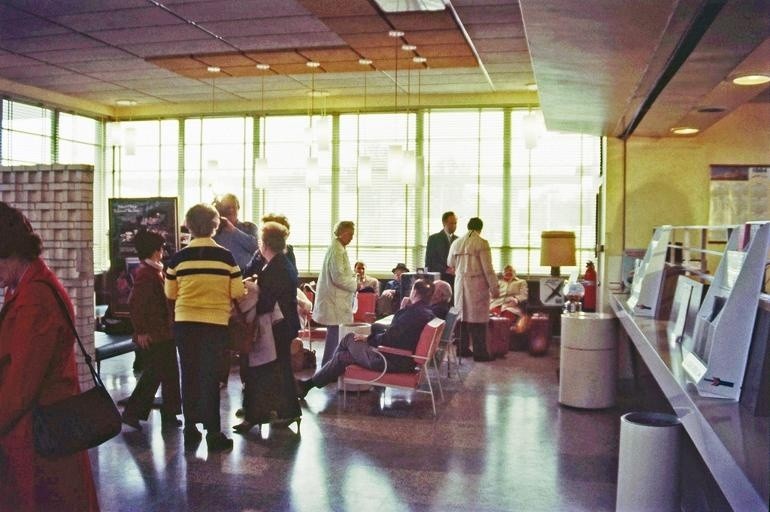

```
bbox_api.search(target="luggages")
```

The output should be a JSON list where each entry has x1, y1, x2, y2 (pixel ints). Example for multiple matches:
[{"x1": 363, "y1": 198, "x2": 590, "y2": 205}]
[
  {"x1": 528, "y1": 312, "x2": 551, "y2": 354},
  {"x1": 485, "y1": 313, "x2": 510, "y2": 358}
]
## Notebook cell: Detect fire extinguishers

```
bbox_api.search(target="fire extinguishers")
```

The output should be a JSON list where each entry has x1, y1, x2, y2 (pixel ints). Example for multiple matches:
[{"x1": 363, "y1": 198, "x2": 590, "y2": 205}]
[{"x1": 583, "y1": 260, "x2": 596, "y2": 309}]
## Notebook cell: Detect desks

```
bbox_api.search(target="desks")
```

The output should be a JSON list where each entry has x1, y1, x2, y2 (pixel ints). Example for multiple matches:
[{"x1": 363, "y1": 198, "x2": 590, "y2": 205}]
[{"x1": 93, "y1": 329, "x2": 162, "y2": 414}]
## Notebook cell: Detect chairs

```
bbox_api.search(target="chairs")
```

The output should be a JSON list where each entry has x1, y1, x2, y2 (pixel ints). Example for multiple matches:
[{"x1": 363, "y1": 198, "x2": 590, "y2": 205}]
[
  {"x1": 426, "y1": 303, "x2": 465, "y2": 403},
  {"x1": 340, "y1": 314, "x2": 448, "y2": 411}
]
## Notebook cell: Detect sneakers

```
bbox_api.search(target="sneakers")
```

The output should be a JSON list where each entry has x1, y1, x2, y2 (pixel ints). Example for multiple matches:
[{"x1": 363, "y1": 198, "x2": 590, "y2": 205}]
[
  {"x1": 121, "y1": 412, "x2": 142, "y2": 431},
  {"x1": 162, "y1": 417, "x2": 182, "y2": 430}
]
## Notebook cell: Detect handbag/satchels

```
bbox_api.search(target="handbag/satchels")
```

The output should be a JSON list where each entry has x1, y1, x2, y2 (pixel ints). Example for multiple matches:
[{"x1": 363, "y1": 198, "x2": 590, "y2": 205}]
[
  {"x1": 32, "y1": 385, "x2": 122, "y2": 456},
  {"x1": 227, "y1": 315, "x2": 258, "y2": 355}
]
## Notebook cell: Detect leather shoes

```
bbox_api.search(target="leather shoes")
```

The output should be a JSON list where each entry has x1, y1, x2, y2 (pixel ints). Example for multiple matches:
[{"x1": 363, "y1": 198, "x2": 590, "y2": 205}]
[
  {"x1": 294, "y1": 377, "x2": 308, "y2": 400},
  {"x1": 456, "y1": 348, "x2": 473, "y2": 357},
  {"x1": 473, "y1": 352, "x2": 496, "y2": 361}
]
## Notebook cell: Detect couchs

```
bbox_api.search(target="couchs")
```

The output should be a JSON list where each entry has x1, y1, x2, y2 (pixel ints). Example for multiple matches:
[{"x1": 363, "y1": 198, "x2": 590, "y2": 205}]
[{"x1": 296, "y1": 277, "x2": 541, "y2": 352}]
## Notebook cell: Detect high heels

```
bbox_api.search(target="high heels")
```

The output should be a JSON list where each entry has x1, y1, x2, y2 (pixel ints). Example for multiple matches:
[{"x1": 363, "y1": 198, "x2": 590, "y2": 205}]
[
  {"x1": 271, "y1": 416, "x2": 302, "y2": 429},
  {"x1": 206, "y1": 433, "x2": 232, "y2": 453},
  {"x1": 231, "y1": 419, "x2": 262, "y2": 432},
  {"x1": 183, "y1": 426, "x2": 201, "y2": 451}
]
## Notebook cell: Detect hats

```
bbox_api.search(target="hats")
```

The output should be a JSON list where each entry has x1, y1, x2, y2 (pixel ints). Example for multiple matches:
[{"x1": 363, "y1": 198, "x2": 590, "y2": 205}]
[{"x1": 392, "y1": 263, "x2": 409, "y2": 272}]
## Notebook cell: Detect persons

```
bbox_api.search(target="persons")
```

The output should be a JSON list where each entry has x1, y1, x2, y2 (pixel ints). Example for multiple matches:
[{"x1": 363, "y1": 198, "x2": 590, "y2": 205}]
[
  {"x1": 120, "y1": 230, "x2": 183, "y2": 430},
  {"x1": 212, "y1": 193, "x2": 258, "y2": 392},
  {"x1": 489, "y1": 265, "x2": 529, "y2": 330},
  {"x1": 0, "y1": 201, "x2": 98, "y2": 511},
  {"x1": 312, "y1": 221, "x2": 364, "y2": 381},
  {"x1": 371, "y1": 280, "x2": 453, "y2": 333},
  {"x1": 295, "y1": 280, "x2": 436, "y2": 399},
  {"x1": 424, "y1": 212, "x2": 459, "y2": 306},
  {"x1": 164, "y1": 203, "x2": 247, "y2": 453},
  {"x1": 231, "y1": 213, "x2": 302, "y2": 437},
  {"x1": 447, "y1": 218, "x2": 500, "y2": 361},
  {"x1": 356, "y1": 261, "x2": 411, "y2": 320}
]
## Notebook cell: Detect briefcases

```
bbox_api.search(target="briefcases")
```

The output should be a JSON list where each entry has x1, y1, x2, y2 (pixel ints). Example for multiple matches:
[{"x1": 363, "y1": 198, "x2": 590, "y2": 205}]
[{"x1": 400, "y1": 267, "x2": 441, "y2": 299}]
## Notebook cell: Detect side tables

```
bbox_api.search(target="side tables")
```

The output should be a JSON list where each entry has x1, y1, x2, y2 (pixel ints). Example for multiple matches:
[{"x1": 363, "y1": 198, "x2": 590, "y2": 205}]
[{"x1": 532, "y1": 302, "x2": 565, "y2": 338}]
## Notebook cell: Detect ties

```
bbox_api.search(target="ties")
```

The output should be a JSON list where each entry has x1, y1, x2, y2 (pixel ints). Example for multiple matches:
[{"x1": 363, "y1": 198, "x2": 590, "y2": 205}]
[{"x1": 449, "y1": 235, "x2": 452, "y2": 243}]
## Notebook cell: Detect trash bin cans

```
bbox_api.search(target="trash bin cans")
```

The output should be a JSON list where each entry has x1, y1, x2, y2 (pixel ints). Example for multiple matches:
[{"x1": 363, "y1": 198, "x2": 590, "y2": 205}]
[{"x1": 615, "y1": 411, "x2": 683, "y2": 512}]
